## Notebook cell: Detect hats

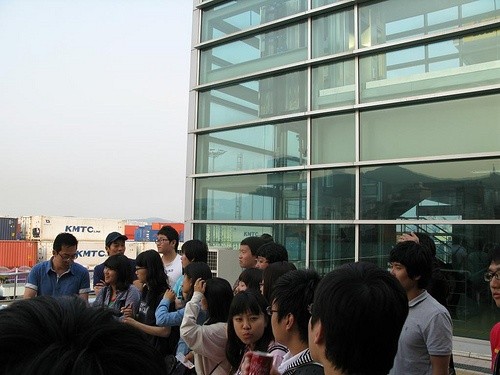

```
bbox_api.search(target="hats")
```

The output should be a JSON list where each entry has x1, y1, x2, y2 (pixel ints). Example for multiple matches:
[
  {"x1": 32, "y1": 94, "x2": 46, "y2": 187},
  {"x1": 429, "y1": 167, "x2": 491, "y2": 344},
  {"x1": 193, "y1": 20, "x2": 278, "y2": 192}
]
[{"x1": 105, "y1": 232, "x2": 128, "y2": 246}]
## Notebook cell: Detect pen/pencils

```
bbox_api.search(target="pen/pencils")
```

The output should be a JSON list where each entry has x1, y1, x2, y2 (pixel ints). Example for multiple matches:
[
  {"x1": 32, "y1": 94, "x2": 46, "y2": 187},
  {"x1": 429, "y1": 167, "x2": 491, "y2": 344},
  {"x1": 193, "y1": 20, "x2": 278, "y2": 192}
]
[{"x1": 120, "y1": 303, "x2": 131, "y2": 313}]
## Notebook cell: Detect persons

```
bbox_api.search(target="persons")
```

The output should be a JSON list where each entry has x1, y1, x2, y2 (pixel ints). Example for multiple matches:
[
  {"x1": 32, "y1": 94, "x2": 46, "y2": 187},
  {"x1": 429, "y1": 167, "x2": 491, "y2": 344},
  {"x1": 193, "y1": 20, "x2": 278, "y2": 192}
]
[
  {"x1": 0, "y1": 226, "x2": 456, "y2": 375},
  {"x1": 484, "y1": 248, "x2": 500, "y2": 375}
]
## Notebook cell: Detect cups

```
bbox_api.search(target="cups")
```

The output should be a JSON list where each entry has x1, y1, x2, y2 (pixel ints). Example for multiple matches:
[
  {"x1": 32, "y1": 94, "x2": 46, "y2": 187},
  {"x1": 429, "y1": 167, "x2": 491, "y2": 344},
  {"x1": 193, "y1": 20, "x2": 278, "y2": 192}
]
[{"x1": 249, "y1": 351, "x2": 274, "y2": 375}]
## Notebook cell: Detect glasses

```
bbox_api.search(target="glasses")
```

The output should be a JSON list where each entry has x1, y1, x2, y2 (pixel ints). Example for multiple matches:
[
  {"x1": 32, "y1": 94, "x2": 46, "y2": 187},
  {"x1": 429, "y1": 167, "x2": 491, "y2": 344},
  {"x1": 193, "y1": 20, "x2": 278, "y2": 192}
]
[
  {"x1": 155, "y1": 238, "x2": 170, "y2": 245},
  {"x1": 266, "y1": 306, "x2": 279, "y2": 315},
  {"x1": 56, "y1": 249, "x2": 78, "y2": 262},
  {"x1": 259, "y1": 282, "x2": 264, "y2": 288},
  {"x1": 134, "y1": 268, "x2": 145, "y2": 272},
  {"x1": 484, "y1": 269, "x2": 500, "y2": 282},
  {"x1": 308, "y1": 303, "x2": 313, "y2": 315}
]
